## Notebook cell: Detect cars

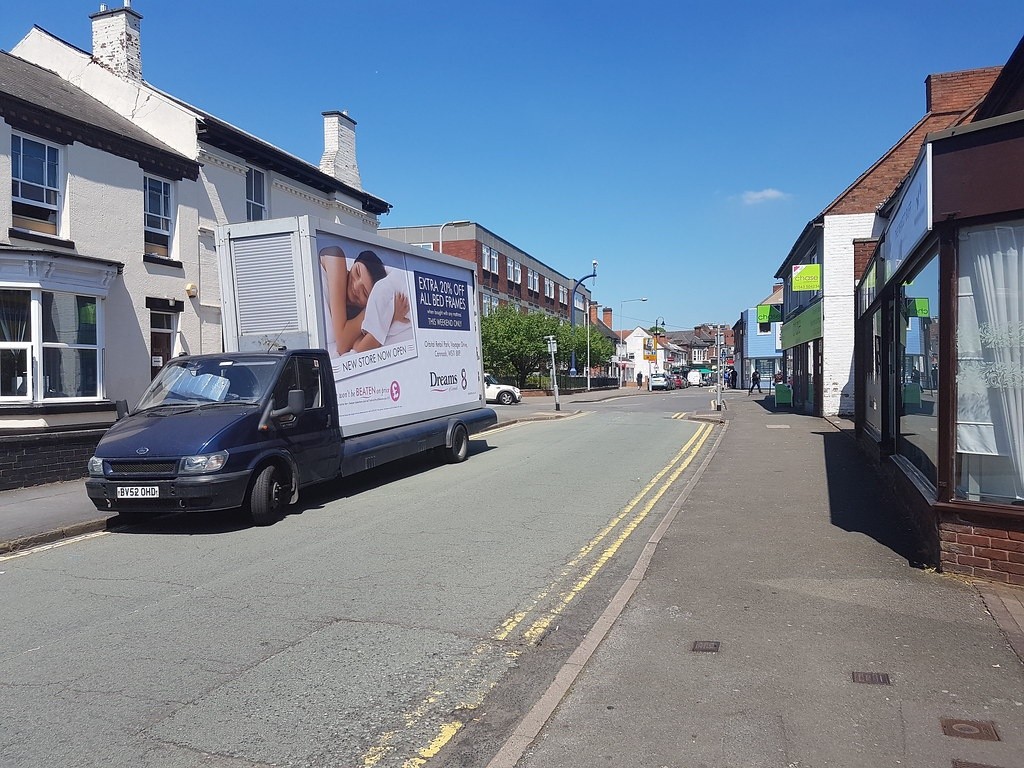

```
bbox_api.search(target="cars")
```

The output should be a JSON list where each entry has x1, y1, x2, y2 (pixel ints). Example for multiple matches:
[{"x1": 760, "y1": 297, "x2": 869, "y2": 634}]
[
  {"x1": 482, "y1": 372, "x2": 523, "y2": 405},
  {"x1": 648, "y1": 372, "x2": 714, "y2": 391}
]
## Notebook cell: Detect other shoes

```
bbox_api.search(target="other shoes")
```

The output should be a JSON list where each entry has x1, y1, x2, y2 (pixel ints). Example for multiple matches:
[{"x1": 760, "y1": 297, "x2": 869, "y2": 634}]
[
  {"x1": 750, "y1": 391, "x2": 753, "y2": 393},
  {"x1": 759, "y1": 392, "x2": 762, "y2": 393}
]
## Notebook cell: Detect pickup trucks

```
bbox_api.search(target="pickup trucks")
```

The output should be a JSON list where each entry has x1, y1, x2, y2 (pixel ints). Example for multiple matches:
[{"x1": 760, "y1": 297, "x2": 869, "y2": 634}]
[{"x1": 81, "y1": 348, "x2": 502, "y2": 526}]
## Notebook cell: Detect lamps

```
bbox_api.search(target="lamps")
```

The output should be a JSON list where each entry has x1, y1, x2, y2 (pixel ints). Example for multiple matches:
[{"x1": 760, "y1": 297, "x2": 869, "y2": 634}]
[{"x1": 169, "y1": 298, "x2": 175, "y2": 306}]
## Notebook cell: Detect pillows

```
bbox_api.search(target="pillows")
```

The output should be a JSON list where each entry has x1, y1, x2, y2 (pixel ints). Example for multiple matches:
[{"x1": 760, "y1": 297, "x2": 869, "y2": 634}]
[{"x1": 360, "y1": 269, "x2": 412, "y2": 345}]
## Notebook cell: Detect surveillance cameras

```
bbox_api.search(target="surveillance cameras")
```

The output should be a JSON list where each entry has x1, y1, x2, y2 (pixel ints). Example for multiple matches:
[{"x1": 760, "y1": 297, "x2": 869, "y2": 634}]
[{"x1": 592, "y1": 260, "x2": 598, "y2": 268}]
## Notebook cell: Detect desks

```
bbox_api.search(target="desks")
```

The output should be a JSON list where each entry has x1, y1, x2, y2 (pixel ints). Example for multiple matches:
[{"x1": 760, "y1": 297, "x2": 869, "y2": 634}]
[
  {"x1": 775, "y1": 384, "x2": 793, "y2": 407},
  {"x1": 901, "y1": 383, "x2": 922, "y2": 408}
]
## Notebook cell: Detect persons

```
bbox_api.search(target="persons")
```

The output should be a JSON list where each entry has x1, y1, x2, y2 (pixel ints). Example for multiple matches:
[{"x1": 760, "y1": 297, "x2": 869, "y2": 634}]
[
  {"x1": 319, "y1": 239, "x2": 411, "y2": 357},
  {"x1": 931, "y1": 367, "x2": 938, "y2": 388},
  {"x1": 731, "y1": 368, "x2": 737, "y2": 389},
  {"x1": 724, "y1": 372, "x2": 731, "y2": 386},
  {"x1": 910, "y1": 365, "x2": 925, "y2": 393},
  {"x1": 637, "y1": 371, "x2": 642, "y2": 390},
  {"x1": 750, "y1": 368, "x2": 763, "y2": 393}
]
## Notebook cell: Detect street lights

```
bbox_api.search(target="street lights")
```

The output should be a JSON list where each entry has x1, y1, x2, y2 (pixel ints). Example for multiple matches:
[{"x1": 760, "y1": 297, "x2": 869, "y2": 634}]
[
  {"x1": 654, "y1": 316, "x2": 665, "y2": 376},
  {"x1": 439, "y1": 219, "x2": 472, "y2": 254},
  {"x1": 619, "y1": 297, "x2": 648, "y2": 388}
]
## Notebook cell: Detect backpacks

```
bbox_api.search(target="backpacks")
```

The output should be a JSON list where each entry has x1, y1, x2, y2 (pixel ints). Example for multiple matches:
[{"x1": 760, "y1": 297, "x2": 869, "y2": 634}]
[
  {"x1": 753, "y1": 372, "x2": 759, "y2": 381},
  {"x1": 724, "y1": 373, "x2": 728, "y2": 381}
]
